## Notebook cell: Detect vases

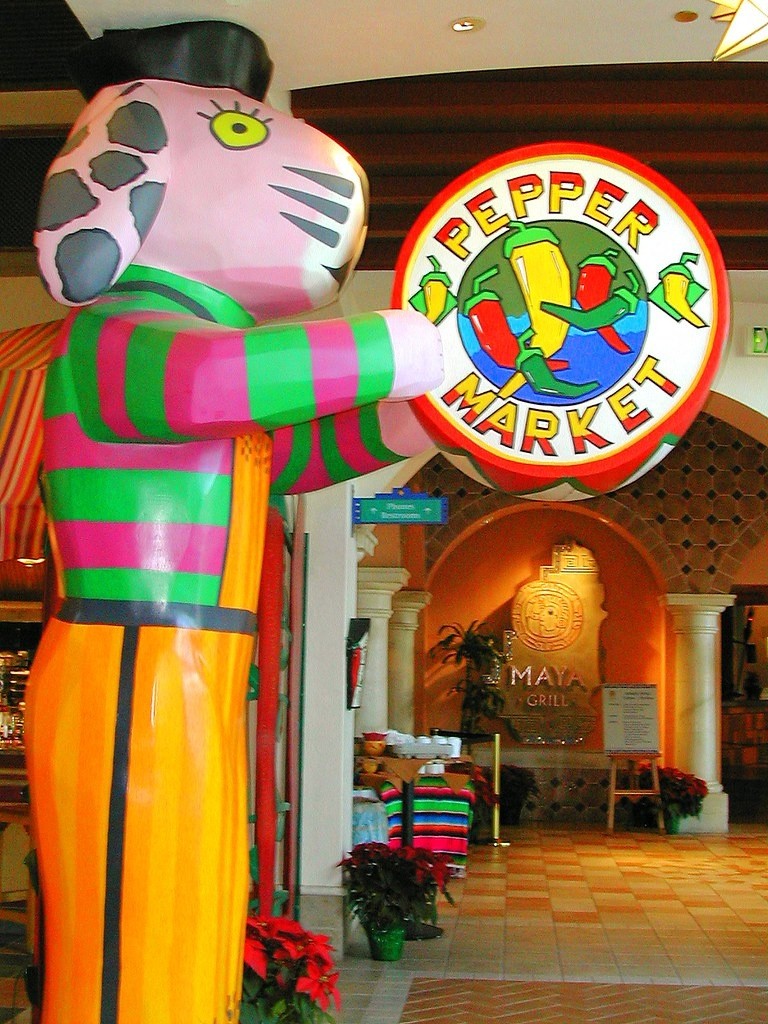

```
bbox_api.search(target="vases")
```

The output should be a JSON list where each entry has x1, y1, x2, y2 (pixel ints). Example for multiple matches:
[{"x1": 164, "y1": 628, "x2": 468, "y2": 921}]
[
  {"x1": 500, "y1": 800, "x2": 525, "y2": 824},
  {"x1": 666, "y1": 803, "x2": 685, "y2": 835},
  {"x1": 631, "y1": 802, "x2": 655, "y2": 828},
  {"x1": 362, "y1": 917, "x2": 407, "y2": 961}
]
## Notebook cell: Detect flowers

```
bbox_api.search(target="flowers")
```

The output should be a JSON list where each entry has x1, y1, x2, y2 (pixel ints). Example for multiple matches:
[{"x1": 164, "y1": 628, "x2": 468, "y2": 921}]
[
  {"x1": 501, "y1": 764, "x2": 537, "y2": 811},
  {"x1": 655, "y1": 768, "x2": 709, "y2": 819},
  {"x1": 335, "y1": 839, "x2": 456, "y2": 929},
  {"x1": 239, "y1": 909, "x2": 344, "y2": 1024},
  {"x1": 615, "y1": 763, "x2": 655, "y2": 804},
  {"x1": 464, "y1": 765, "x2": 497, "y2": 829}
]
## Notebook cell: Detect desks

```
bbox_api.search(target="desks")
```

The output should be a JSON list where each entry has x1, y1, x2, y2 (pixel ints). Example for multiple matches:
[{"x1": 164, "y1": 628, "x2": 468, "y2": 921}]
[{"x1": 354, "y1": 756, "x2": 470, "y2": 940}]
